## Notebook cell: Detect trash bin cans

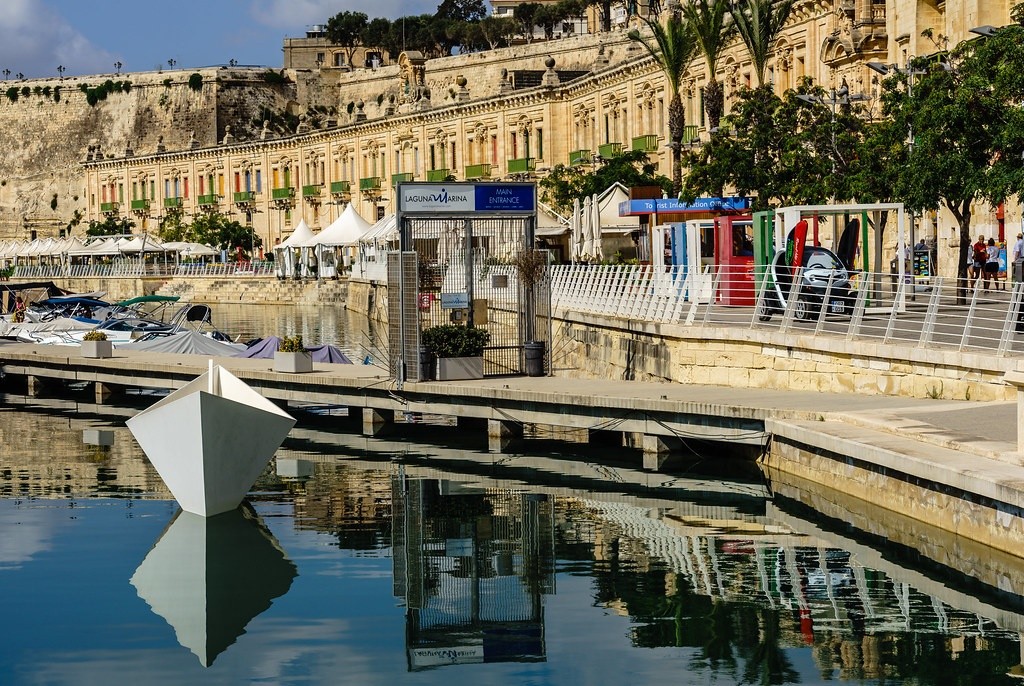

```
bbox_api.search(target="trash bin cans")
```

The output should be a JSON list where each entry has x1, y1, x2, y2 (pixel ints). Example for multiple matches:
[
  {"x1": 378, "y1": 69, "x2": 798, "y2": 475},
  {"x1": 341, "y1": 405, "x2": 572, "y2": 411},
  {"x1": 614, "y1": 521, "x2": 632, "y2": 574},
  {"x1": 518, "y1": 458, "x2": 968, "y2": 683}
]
[
  {"x1": 890, "y1": 258, "x2": 915, "y2": 301},
  {"x1": 524, "y1": 341, "x2": 546, "y2": 377}
]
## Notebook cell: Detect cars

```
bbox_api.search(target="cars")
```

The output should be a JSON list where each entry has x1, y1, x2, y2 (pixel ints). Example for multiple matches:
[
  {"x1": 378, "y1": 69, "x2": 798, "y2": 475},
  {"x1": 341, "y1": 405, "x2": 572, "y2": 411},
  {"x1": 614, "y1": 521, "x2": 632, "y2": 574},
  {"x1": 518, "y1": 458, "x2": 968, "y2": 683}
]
[{"x1": 755, "y1": 218, "x2": 866, "y2": 321}]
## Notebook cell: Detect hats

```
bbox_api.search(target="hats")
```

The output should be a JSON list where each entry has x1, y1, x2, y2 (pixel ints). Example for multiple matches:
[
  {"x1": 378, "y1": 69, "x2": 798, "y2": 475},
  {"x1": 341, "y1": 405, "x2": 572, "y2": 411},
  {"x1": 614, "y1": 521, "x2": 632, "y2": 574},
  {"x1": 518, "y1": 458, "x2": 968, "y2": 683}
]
[{"x1": 1016, "y1": 233, "x2": 1023, "y2": 239}]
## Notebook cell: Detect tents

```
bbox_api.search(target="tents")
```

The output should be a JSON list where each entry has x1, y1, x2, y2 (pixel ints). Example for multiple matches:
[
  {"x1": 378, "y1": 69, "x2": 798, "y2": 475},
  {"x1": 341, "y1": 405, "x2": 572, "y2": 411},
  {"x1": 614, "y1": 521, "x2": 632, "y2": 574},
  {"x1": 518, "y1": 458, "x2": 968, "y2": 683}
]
[
  {"x1": 274, "y1": 217, "x2": 316, "y2": 279},
  {"x1": 356, "y1": 180, "x2": 571, "y2": 296},
  {"x1": 591, "y1": 183, "x2": 655, "y2": 265},
  {"x1": 304, "y1": 202, "x2": 372, "y2": 280},
  {"x1": 179, "y1": 243, "x2": 218, "y2": 266},
  {"x1": 0, "y1": 236, "x2": 168, "y2": 276}
]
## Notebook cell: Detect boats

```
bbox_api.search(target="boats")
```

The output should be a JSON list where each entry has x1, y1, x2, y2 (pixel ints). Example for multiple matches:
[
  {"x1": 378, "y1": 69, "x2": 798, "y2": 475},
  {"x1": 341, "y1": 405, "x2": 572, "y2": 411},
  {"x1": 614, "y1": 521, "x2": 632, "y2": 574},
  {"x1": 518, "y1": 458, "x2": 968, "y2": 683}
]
[
  {"x1": 124, "y1": 360, "x2": 297, "y2": 517},
  {"x1": 129, "y1": 499, "x2": 299, "y2": 669},
  {"x1": 1, "y1": 281, "x2": 356, "y2": 365}
]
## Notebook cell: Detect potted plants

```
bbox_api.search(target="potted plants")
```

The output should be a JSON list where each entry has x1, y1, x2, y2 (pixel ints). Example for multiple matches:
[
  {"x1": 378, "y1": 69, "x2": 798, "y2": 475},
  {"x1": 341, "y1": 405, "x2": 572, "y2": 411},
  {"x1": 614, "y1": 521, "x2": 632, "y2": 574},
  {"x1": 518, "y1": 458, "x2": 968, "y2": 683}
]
[
  {"x1": 274, "y1": 334, "x2": 313, "y2": 373},
  {"x1": 419, "y1": 323, "x2": 491, "y2": 380},
  {"x1": 82, "y1": 329, "x2": 112, "y2": 358}
]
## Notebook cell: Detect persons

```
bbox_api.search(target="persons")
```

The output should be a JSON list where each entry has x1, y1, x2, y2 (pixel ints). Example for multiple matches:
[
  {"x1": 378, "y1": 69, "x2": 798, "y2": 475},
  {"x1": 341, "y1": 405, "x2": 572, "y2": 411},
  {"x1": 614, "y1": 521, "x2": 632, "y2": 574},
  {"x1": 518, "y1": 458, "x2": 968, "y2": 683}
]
[
  {"x1": 914, "y1": 239, "x2": 928, "y2": 250},
  {"x1": 15, "y1": 297, "x2": 26, "y2": 323},
  {"x1": 972, "y1": 235, "x2": 987, "y2": 285},
  {"x1": 1012, "y1": 233, "x2": 1024, "y2": 261},
  {"x1": 984, "y1": 238, "x2": 1000, "y2": 293},
  {"x1": 896, "y1": 243, "x2": 909, "y2": 278},
  {"x1": 967, "y1": 236, "x2": 975, "y2": 293}
]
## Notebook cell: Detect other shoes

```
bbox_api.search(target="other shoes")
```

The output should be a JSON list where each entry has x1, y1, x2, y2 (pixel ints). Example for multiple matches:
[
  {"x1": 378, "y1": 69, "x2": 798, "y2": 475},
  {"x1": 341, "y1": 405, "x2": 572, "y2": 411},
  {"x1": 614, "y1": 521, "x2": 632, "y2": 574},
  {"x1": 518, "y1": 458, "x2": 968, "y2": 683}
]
[{"x1": 969, "y1": 290, "x2": 974, "y2": 293}]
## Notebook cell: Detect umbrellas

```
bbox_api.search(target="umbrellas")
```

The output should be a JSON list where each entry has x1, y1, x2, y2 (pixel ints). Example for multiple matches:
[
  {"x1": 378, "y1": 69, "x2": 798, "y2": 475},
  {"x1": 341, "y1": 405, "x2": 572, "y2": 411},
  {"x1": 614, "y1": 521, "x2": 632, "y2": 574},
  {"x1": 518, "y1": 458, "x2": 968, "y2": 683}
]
[
  {"x1": 590, "y1": 194, "x2": 604, "y2": 265},
  {"x1": 436, "y1": 220, "x2": 465, "y2": 265},
  {"x1": 581, "y1": 196, "x2": 594, "y2": 265},
  {"x1": 572, "y1": 198, "x2": 582, "y2": 265},
  {"x1": 504, "y1": 220, "x2": 513, "y2": 264},
  {"x1": 496, "y1": 220, "x2": 505, "y2": 265},
  {"x1": 515, "y1": 221, "x2": 525, "y2": 263}
]
